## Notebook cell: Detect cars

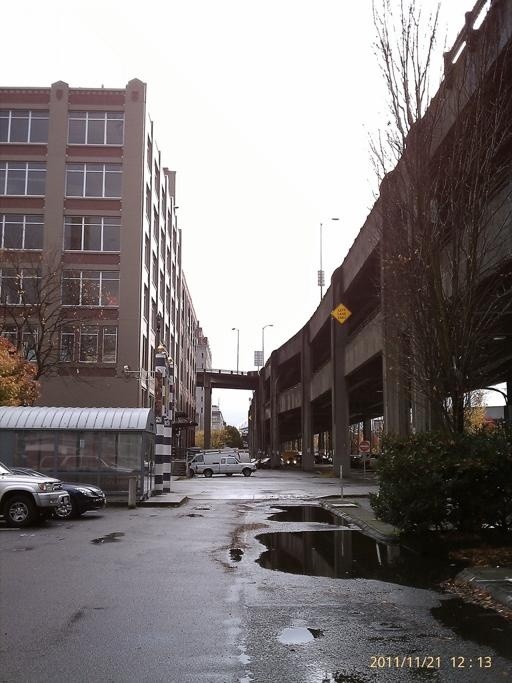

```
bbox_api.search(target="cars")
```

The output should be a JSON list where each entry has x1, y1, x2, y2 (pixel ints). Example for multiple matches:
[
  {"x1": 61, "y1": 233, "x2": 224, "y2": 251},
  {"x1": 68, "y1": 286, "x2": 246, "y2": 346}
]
[
  {"x1": 251, "y1": 458, "x2": 271, "y2": 469},
  {"x1": 287, "y1": 454, "x2": 331, "y2": 465},
  {"x1": 11, "y1": 467, "x2": 107, "y2": 520}
]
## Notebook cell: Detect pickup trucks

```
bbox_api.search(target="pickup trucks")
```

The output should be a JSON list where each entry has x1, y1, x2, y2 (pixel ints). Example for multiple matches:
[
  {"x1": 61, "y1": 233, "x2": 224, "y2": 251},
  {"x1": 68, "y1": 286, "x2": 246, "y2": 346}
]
[{"x1": 196, "y1": 456, "x2": 256, "y2": 477}]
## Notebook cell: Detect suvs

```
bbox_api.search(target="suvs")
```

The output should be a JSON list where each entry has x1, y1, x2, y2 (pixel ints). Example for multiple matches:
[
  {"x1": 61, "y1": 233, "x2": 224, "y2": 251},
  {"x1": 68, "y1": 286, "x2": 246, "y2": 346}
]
[
  {"x1": 40, "y1": 455, "x2": 133, "y2": 491},
  {"x1": 0, "y1": 460, "x2": 71, "y2": 528}
]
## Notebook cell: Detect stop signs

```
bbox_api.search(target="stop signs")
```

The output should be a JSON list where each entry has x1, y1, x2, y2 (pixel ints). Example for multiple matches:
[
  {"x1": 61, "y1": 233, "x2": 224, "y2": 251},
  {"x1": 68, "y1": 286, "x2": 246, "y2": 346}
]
[{"x1": 358, "y1": 441, "x2": 370, "y2": 452}]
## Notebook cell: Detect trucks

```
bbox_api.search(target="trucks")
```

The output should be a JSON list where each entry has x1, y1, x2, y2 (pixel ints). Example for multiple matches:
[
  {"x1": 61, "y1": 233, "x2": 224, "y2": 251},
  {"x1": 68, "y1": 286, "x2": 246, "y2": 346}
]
[{"x1": 188, "y1": 448, "x2": 240, "y2": 477}]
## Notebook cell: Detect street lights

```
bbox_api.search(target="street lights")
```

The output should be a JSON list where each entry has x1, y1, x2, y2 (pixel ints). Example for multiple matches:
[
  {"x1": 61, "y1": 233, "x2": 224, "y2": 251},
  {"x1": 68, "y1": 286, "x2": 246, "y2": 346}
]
[
  {"x1": 262, "y1": 324, "x2": 273, "y2": 367},
  {"x1": 318, "y1": 216, "x2": 339, "y2": 299},
  {"x1": 232, "y1": 328, "x2": 240, "y2": 374}
]
